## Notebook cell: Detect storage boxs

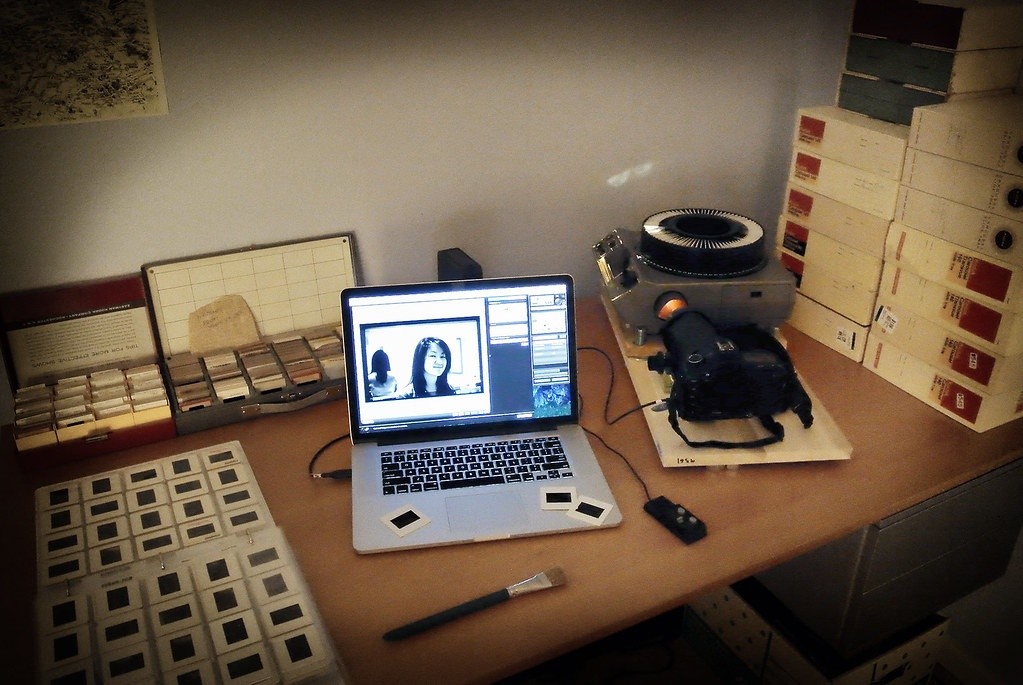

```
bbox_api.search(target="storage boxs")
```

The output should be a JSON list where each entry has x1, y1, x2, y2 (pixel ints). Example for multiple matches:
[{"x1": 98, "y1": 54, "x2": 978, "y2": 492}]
[{"x1": 774, "y1": 3, "x2": 1023, "y2": 434}]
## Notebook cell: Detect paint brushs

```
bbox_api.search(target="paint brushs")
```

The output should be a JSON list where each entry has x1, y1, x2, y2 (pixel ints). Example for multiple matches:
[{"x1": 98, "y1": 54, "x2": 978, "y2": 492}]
[{"x1": 381, "y1": 565, "x2": 570, "y2": 641}]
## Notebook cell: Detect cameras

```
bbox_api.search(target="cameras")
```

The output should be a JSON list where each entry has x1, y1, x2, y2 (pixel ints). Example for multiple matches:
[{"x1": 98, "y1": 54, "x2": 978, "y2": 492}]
[{"x1": 659, "y1": 306, "x2": 793, "y2": 422}]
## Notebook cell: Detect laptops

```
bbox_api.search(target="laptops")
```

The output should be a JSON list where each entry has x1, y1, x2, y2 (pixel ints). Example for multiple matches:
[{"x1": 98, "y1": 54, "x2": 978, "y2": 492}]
[{"x1": 341, "y1": 274, "x2": 623, "y2": 555}]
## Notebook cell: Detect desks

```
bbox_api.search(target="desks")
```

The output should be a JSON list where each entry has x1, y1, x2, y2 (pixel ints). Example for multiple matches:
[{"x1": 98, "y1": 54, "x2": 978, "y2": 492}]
[{"x1": 1, "y1": 298, "x2": 1023, "y2": 685}]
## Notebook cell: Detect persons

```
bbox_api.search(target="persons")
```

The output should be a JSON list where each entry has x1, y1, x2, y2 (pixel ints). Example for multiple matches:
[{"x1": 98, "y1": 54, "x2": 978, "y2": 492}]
[
  {"x1": 396, "y1": 336, "x2": 454, "y2": 399},
  {"x1": 367, "y1": 350, "x2": 398, "y2": 396}
]
